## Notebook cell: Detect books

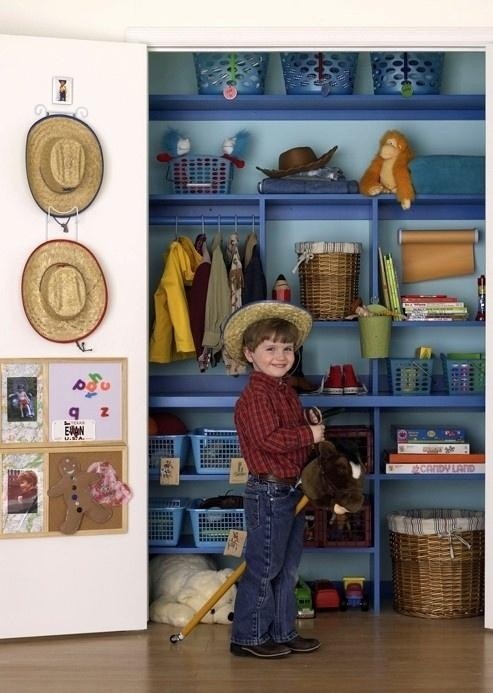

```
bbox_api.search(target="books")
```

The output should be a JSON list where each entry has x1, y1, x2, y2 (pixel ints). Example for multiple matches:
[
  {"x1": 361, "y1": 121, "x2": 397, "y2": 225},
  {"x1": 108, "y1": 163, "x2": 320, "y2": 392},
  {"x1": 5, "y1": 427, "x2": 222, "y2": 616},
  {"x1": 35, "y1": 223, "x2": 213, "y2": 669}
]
[
  {"x1": 400, "y1": 293, "x2": 469, "y2": 322},
  {"x1": 378, "y1": 245, "x2": 403, "y2": 321}
]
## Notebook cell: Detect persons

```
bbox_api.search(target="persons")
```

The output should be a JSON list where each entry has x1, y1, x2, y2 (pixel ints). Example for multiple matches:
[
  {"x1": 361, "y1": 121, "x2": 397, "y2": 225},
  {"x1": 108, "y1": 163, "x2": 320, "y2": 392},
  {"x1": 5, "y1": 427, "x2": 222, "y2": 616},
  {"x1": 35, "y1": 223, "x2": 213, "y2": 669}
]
[
  {"x1": 16, "y1": 470, "x2": 37, "y2": 498},
  {"x1": 8, "y1": 385, "x2": 32, "y2": 418},
  {"x1": 226, "y1": 302, "x2": 325, "y2": 659}
]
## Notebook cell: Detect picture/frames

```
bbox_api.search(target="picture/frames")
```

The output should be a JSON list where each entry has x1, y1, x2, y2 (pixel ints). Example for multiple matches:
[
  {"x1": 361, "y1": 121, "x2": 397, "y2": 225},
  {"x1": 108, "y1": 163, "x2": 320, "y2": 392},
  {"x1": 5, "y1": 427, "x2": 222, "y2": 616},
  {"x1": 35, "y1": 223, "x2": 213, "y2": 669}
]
[
  {"x1": 52, "y1": 76, "x2": 73, "y2": 105},
  {"x1": 0, "y1": 448, "x2": 132, "y2": 540},
  {"x1": 0, "y1": 356, "x2": 128, "y2": 450}
]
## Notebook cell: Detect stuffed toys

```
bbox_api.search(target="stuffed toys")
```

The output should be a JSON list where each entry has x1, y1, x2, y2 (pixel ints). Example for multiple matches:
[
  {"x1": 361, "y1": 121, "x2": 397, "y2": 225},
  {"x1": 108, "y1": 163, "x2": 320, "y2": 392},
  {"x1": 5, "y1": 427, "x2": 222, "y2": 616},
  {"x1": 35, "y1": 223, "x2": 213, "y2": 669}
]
[
  {"x1": 204, "y1": 130, "x2": 249, "y2": 186},
  {"x1": 155, "y1": 127, "x2": 193, "y2": 187},
  {"x1": 299, "y1": 406, "x2": 363, "y2": 515},
  {"x1": 148, "y1": 556, "x2": 237, "y2": 627},
  {"x1": 344, "y1": 298, "x2": 408, "y2": 320},
  {"x1": 358, "y1": 131, "x2": 413, "y2": 209}
]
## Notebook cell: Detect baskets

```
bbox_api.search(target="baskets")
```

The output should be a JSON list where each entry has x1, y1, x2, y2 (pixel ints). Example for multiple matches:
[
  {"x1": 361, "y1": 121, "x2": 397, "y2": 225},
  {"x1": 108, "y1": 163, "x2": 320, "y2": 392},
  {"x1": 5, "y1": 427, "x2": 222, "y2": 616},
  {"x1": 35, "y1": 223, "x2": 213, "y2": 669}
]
[
  {"x1": 387, "y1": 508, "x2": 485, "y2": 619},
  {"x1": 295, "y1": 241, "x2": 362, "y2": 321}
]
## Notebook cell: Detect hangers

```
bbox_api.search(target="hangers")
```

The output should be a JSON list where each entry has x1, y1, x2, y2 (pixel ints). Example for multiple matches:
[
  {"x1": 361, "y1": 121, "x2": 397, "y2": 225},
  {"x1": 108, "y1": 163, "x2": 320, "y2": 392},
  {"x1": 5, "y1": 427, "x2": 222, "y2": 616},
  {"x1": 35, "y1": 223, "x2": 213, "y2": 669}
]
[
  {"x1": 230, "y1": 215, "x2": 238, "y2": 262},
  {"x1": 195, "y1": 215, "x2": 210, "y2": 269},
  {"x1": 212, "y1": 215, "x2": 223, "y2": 270},
  {"x1": 163, "y1": 217, "x2": 185, "y2": 272},
  {"x1": 245, "y1": 214, "x2": 260, "y2": 259}
]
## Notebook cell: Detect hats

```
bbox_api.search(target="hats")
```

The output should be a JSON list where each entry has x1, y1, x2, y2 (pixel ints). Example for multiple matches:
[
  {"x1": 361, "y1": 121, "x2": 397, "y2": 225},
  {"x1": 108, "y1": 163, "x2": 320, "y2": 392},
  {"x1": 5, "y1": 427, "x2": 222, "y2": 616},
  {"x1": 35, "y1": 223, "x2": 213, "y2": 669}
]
[
  {"x1": 255, "y1": 145, "x2": 341, "y2": 175},
  {"x1": 26, "y1": 114, "x2": 103, "y2": 217},
  {"x1": 222, "y1": 302, "x2": 312, "y2": 367},
  {"x1": 21, "y1": 239, "x2": 109, "y2": 344}
]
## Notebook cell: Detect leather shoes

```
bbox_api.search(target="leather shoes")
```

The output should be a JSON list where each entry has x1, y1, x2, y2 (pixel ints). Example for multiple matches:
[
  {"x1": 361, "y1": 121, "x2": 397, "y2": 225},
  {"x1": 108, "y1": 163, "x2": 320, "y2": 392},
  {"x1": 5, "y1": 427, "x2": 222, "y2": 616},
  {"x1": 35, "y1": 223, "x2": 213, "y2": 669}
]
[
  {"x1": 231, "y1": 638, "x2": 290, "y2": 658},
  {"x1": 279, "y1": 635, "x2": 322, "y2": 653}
]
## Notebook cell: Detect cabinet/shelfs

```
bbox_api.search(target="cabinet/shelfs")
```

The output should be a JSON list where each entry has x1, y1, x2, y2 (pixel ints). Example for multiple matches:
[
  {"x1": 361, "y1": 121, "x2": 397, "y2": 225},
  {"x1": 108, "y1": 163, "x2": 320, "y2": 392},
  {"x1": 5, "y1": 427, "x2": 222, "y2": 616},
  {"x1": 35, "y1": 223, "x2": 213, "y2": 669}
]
[{"x1": 149, "y1": 95, "x2": 485, "y2": 615}]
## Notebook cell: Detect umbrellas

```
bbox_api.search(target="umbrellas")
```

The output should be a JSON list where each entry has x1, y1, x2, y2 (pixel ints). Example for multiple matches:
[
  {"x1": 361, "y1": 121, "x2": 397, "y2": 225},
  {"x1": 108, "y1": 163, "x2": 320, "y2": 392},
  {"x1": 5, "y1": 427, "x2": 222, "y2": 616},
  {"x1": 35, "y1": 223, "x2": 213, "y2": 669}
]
[{"x1": 257, "y1": 178, "x2": 359, "y2": 194}]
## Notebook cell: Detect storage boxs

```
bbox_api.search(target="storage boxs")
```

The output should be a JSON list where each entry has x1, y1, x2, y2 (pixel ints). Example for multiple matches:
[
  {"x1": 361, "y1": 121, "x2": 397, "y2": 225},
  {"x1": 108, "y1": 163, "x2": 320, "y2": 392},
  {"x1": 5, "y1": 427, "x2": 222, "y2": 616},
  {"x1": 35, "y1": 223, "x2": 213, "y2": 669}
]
[
  {"x1": 324, "y1": 424, "x2": 372, "y2": 474},
  {"x1": 149, "y1": 435, "x2": 192, "y2": 473},
  {"x1": 370, "y1": 51, "x2": 443, "y2": 95},
  {"x1": 386, "y1": 358, "x2": 432, "y2": 396},
  {"x1": 280, "y1": 53, "x2": 359, "y2": 95},
  {"x1": 188, "y1": 426, "x2": 244, "y2": 473},
  {"x1": 319, "y1": 503, "x2": 372, "y2": 547},
  {"x1": 303, "y1": 504, "x2": 319, "y2": 549},
  {"x1": 166, "y1": 154, "x2": 234, "y2": 194},
  {"x1": 192, "y1": 52, "x2": 268, "y2": 94},
  {"x1": 186, "y1": 499, "x2": 244, "y2": 547},
  {"x1": 441, "y1": 353, "x2": 485, "y2": 396},
  {"x1": 148, "y1": 498, "x2": 187, "y2": 547},
  {"x1": 408, "y1": 155, "x2": 485, "y2": 194}
]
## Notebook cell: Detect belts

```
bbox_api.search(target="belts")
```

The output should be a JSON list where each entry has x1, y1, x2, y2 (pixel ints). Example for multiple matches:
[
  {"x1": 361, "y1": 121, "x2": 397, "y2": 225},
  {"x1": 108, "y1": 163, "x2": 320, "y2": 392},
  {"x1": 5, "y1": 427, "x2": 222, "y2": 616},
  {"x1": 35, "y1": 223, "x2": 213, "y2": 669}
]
[{"x1": 248, "y1": 473, "x2": 299, "y2": 485}]
